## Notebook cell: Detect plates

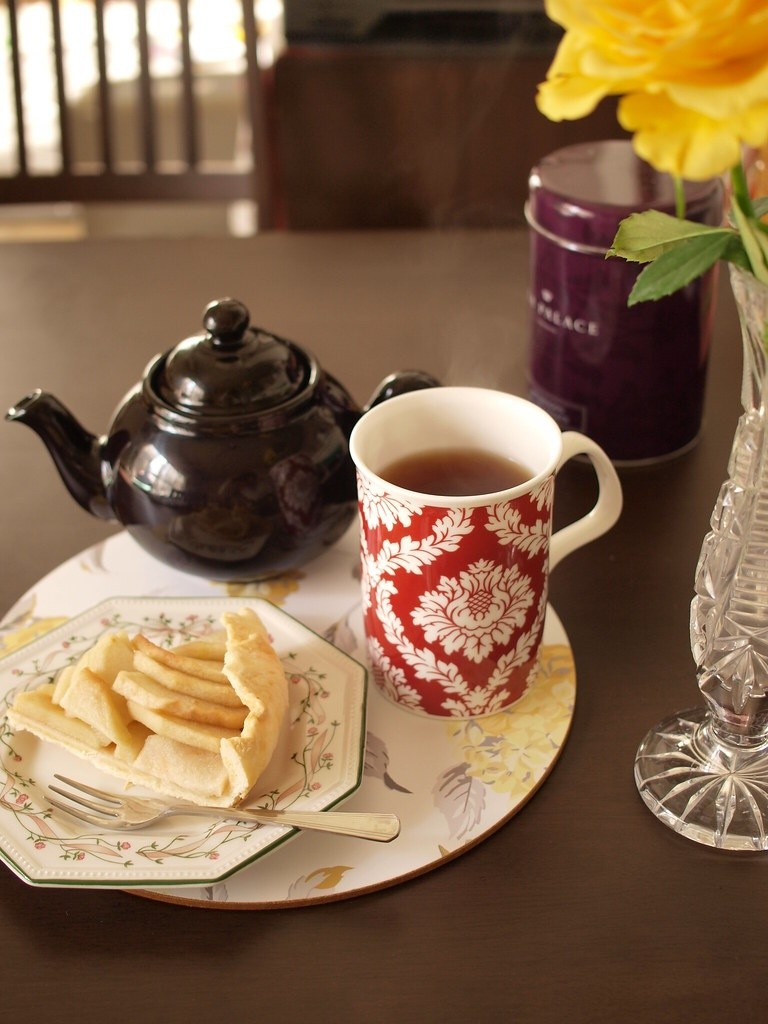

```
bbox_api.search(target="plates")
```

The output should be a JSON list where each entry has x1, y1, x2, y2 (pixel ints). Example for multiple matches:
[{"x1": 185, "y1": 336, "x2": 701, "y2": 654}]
[
  {"x1": 0, "y1": 506, "x2": 577, "y2": 910},
  {"x1": 0, "y1": 596, "x2": 368, "y2": 889}
]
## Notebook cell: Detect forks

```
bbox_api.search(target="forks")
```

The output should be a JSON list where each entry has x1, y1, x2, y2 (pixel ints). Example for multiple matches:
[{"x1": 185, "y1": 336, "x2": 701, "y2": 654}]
[{"x1": 42, "y1": 773, "x2": 400, "y2": 843}]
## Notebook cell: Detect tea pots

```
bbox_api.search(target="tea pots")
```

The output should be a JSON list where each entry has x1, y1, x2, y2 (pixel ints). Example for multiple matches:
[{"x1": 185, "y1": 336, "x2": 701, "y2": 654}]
[{"x1": 6, "y1": 298, "x2": 438, "y2": 584}]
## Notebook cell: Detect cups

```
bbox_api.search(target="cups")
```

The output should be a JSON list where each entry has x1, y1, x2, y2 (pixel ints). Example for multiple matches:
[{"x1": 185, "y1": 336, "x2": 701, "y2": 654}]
[
  {"x1": 348, "y1": 386, "x2": 623, "y2": 720},
  {"x1": 525, "y1": 139, "x2": 727, "y2": 469}
]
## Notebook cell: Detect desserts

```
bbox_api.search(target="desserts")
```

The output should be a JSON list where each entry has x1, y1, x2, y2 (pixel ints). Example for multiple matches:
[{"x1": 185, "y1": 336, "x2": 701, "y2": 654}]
[{"x1": 4, "y1": 604, "x2": 287, "y2": 812}]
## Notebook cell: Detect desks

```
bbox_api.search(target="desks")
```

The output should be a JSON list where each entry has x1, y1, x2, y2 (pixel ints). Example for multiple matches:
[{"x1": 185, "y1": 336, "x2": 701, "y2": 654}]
[{"x1": 0, "y1": 229, "x2": 768, "y2": 1024}]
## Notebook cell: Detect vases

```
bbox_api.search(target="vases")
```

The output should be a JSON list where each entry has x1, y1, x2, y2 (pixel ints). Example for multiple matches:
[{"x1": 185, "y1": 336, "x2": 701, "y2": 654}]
[{"x1": 632, "y1": 261, "x2": 768, "y2": 852}]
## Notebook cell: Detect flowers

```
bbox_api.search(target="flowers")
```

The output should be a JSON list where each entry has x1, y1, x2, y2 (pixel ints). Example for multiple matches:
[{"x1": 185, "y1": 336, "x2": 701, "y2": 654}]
[{"x1": 535, "y1": 0, "x2": 768, "y2": 310}]
